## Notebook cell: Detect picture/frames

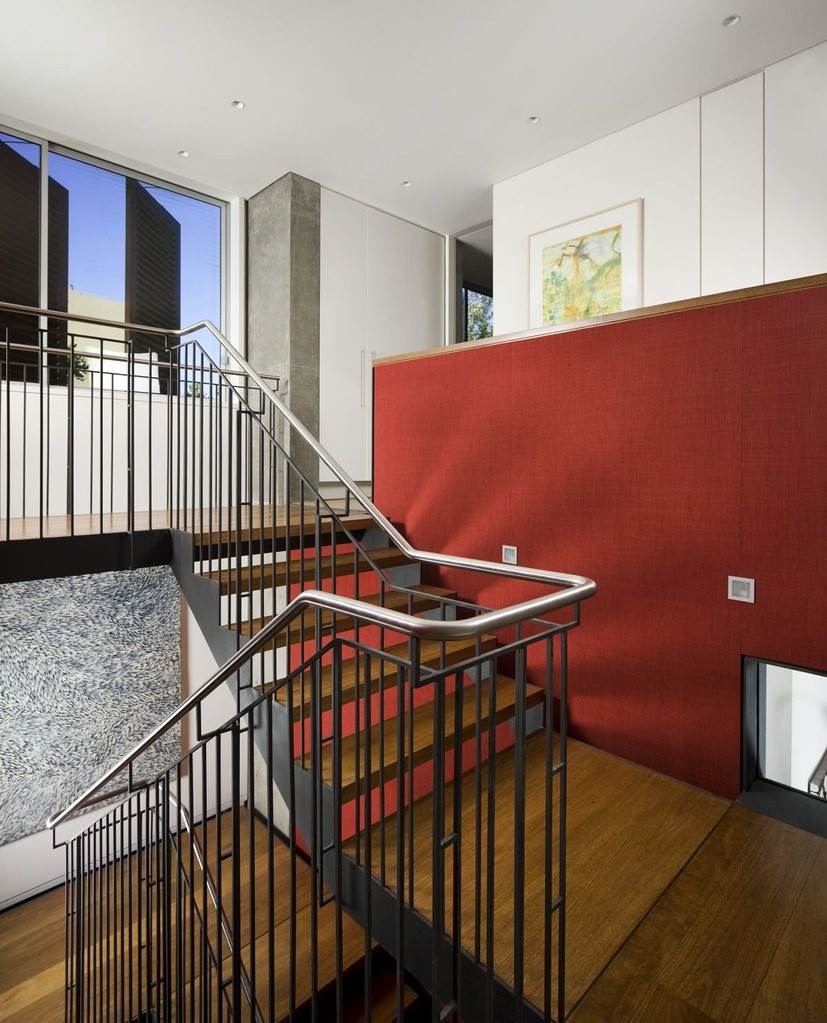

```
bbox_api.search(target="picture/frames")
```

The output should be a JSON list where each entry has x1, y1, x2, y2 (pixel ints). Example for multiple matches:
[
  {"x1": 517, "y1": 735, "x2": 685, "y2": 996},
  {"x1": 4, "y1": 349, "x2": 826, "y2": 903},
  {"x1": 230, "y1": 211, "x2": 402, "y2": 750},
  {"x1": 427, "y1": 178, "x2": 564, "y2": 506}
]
[{"x1": 527, "y1": 198, "x2": 643, "y2": 330}]
